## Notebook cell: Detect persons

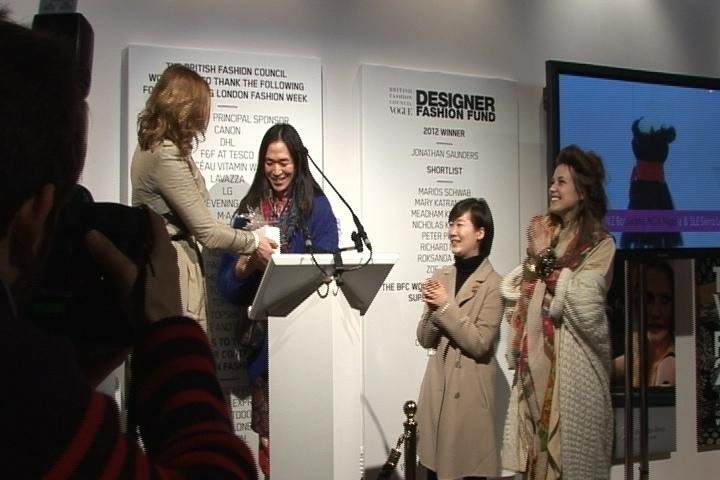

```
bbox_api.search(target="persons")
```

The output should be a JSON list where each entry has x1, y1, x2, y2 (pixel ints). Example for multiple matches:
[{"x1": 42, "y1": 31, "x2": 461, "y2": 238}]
[
  {"x1": 130, "y1": 64, "x2": 278, "y2": 334},
  {"x1": 417, "y1": 197, "x2": 511, "y2": 480},
  {"x1": 218, "y1": 123, "x2": 339, "y2": 480},
  {"x1": 504, "y1": 144, "x2": 616, "y2": 480},
  {"x1": 611, "y1": 265, "x2": 676, "y2": 386},
  {"x1": 0, "y1": 4, "x2": 259, "y2": 480}
]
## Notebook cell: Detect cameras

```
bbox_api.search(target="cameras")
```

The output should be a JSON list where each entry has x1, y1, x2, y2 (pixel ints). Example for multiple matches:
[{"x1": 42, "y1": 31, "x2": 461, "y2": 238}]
[{"x1": 22, "y1": 200, "x2": 148, "y2": 345}]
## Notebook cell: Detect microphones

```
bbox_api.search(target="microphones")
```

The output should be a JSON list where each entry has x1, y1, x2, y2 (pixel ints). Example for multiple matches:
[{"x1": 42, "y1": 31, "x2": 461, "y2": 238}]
[
  {"x1": 304, "y1": 232, "x2": 314, "y2": 253},
  {"x1": 302, "y1": 147, "x2": 373, "y2": 250}
]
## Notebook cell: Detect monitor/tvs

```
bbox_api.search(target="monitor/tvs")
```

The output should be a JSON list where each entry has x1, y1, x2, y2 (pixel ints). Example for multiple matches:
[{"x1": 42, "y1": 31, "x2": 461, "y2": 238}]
[{"x1": 546, "y1": 59, "x2": 720, "y2": 260}]
